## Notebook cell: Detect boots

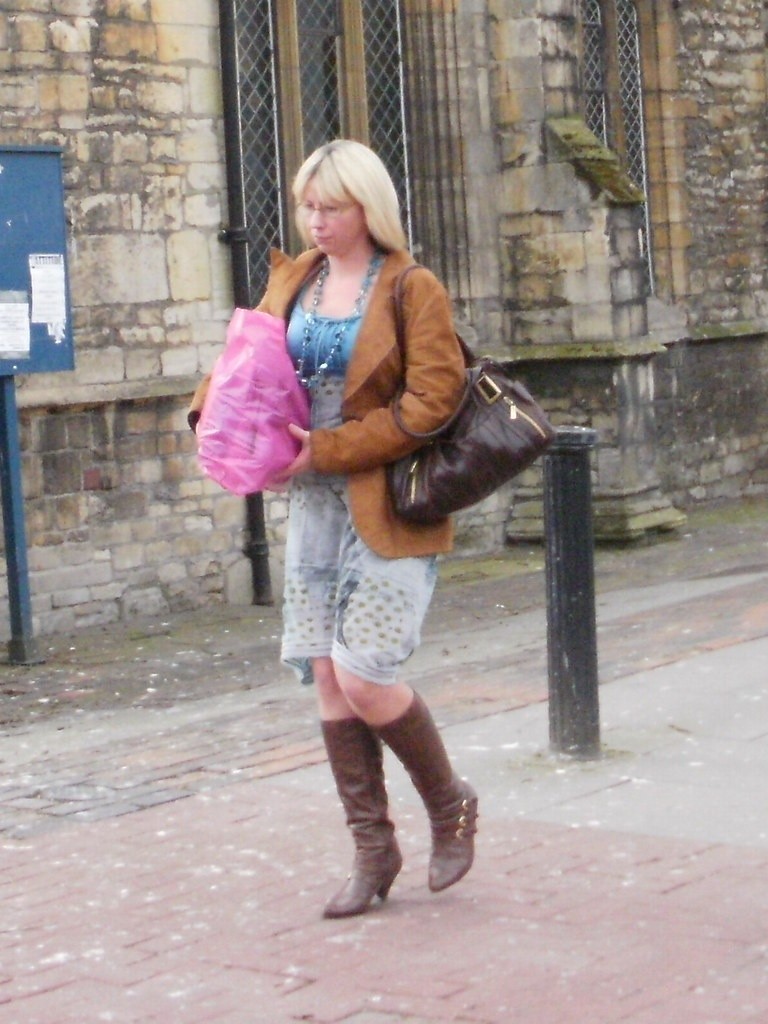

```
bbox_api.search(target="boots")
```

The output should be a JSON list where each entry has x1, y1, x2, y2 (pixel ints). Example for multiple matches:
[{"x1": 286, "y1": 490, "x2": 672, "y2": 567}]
[
  {"x1": 319, "y1": 718, "x2": 405, "y2": 918},
  {"x1": 372, "y1": 687, "x2": 479, "y2": 893}
]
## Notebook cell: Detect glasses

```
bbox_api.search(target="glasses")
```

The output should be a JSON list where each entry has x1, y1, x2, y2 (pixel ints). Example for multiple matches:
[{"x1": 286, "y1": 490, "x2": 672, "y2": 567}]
[{"x1": 303, "y1": 201, "x2": 357, "y2": 215}]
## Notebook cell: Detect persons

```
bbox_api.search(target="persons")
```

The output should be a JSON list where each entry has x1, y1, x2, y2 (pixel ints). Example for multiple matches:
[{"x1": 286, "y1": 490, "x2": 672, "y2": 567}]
[{"x1": 186, "y1": 138, "x2": 479, "y2": 919}]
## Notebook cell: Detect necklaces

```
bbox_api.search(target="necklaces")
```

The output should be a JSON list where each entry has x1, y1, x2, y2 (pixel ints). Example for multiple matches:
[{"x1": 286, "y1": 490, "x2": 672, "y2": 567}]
[{"x1": 296, "y1": 248, "x2": 383, "y2": 390}]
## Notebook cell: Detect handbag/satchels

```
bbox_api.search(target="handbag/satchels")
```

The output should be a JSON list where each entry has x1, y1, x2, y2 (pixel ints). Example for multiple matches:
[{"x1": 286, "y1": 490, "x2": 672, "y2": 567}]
[
  {"x1": 197, "y1": 308, "x2": 310, "y2": 497},
  {"x1": 386, "y1": 262, "x2": 556, "y2": 524}
]
